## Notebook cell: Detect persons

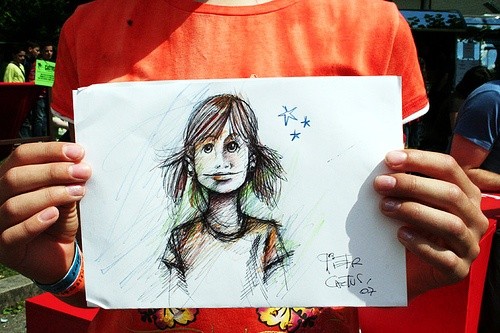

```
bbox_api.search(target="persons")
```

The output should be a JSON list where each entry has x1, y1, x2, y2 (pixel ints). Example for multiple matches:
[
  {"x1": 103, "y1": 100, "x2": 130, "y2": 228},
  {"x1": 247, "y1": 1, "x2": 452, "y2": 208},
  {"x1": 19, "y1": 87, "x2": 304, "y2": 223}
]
[
  {"x1": 0, "y1": 0, "x2": 489, "y2": 333},
  {"x1": 436, "y1": 62, "x2": 500, "y2": 191},
  {"x1": 3, "y1": 41, "x2": 54, "y2": 139}
]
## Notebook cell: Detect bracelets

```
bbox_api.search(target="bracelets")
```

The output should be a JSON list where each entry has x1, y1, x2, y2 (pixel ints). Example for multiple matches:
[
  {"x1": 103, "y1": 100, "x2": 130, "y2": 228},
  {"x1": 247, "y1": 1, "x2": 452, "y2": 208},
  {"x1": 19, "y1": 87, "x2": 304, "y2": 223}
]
[
  {"x1": 55, "y1": 253, "x2": 85, "y2": 297},
  {"x1": 30, "y1": 239, "x2": 82, "y2": 294}
]
